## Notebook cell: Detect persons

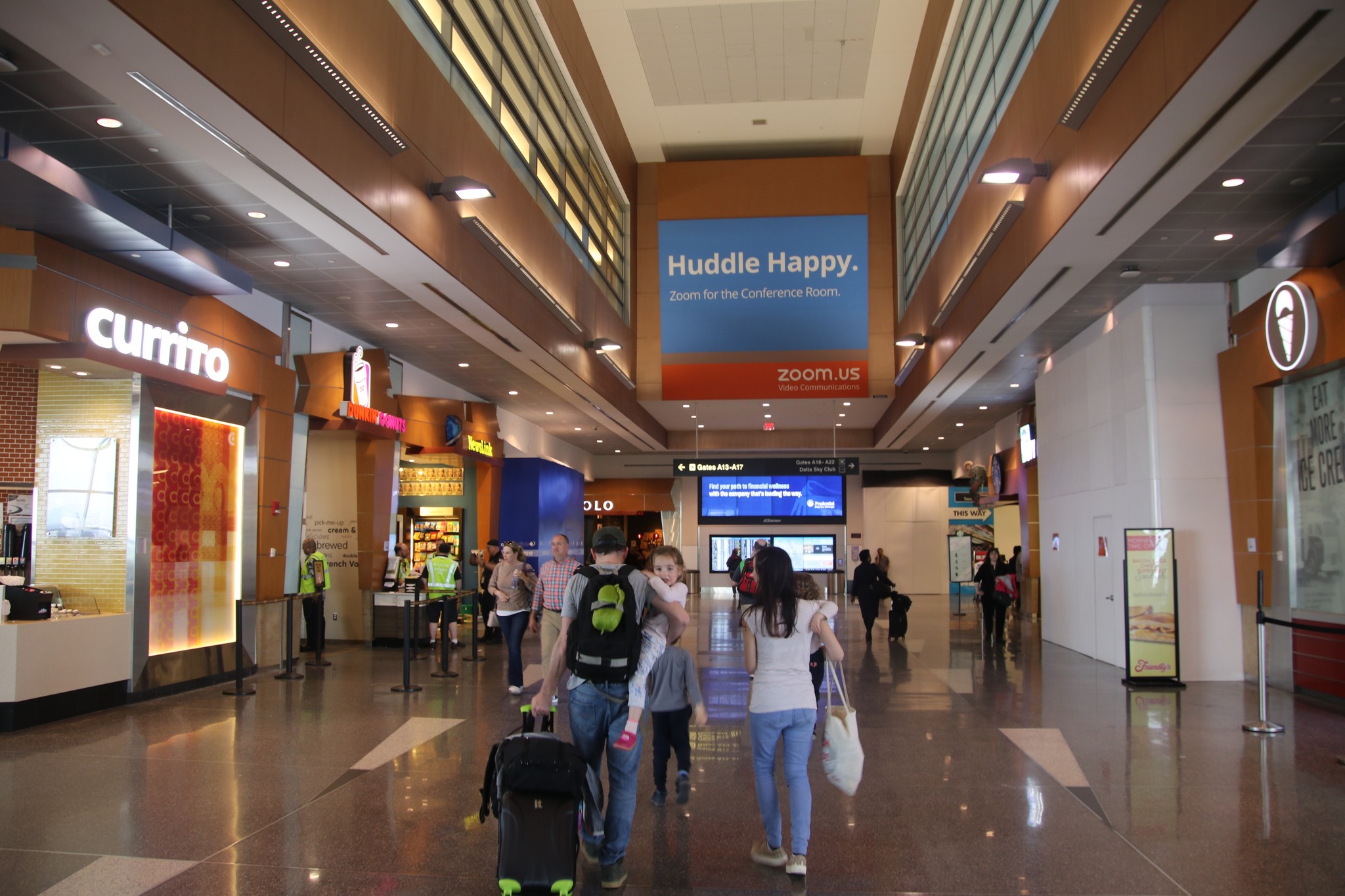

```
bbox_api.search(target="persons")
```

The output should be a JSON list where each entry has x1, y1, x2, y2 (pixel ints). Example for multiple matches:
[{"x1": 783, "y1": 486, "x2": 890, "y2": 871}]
[
  {"x1": 420, "y1": 544, "x2": 468, "y2": 648},
  {"x1": 470, "y1": 540, "x2": 505, "y2": 644},
  {"x1": 791, "y1": 572, "x2": 839, "y2": 738},
  {"x1": 395, "y1": 543, "x2": 421, "y2": 585},
  {"x1": 851, "y1": 546, "x2": 1032, "y2": 653},
  {"x1": 739, "y1": 547, "x2": 844, "y2": 874},
  {"x1": 424, "y1": 539, "x2": 452, "y2": 564},
  {"x1": 531, "y1": 526, "x2": 689, "y2": 887},
  {"x1": 613, "y1": 546, "x2": 688, "y2": 750},
  {"x1": 726, "y1": 549, "x2": 742, "y2": 597},
  {"x1": 645, "y1": 633, "x2": 709, "y2": 806},
  {"x1": 300, "y1": 538, "x2": 330, "y2": 651},
  {"x1": 528, "y1": 534, "x2": 583, "y2": 703},
  {"x1": 733, "y1": 539, "x2": 769, "y2": 680},
  {"x1": 488, "y1": 541, "x2": 537, "y2": 694}
]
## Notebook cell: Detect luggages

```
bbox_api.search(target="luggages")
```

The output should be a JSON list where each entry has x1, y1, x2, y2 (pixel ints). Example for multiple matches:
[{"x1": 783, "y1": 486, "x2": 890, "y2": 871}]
[
  {"x1": 888, "y1": 585, "x2": 908, "y2": 641},
  {"x1": 496, "y1": 703, "x2": 585, "y2": 896}
]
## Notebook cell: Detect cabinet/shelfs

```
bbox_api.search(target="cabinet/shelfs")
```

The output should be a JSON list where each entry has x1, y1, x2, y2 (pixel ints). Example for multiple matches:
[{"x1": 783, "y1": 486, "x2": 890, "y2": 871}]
[{"x1": 373, "y1": 592, "x2": 427, "y2": 639}]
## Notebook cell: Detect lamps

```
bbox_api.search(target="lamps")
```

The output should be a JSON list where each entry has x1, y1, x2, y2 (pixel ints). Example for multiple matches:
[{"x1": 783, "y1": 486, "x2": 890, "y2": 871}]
[
  {"x1": 585, "y1": 338, "x2": 624, "y2": 350},
  {"x1": 977, "y1": 157, "x2": 1052, "y2": 184},
  {"x1": 894, "y1": 333, "x2": 935, "y2": 346},
  {"x1": 427, "y1": 175, "x2": 497, "y2": 202}
]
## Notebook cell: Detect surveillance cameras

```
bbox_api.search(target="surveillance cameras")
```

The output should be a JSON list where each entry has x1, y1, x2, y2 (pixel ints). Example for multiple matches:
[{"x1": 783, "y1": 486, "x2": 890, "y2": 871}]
[
  {"x1": 904, "y1": 450, "x2": 908, "y2": 454},
  {"x1": 594, "y1": 426, "x2": 598, "y2": 431}
]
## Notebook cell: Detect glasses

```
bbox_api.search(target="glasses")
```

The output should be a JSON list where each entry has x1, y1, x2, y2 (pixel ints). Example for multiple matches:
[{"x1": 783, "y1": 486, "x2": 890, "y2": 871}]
[{"x1": 507, "y1": 541, "x2": 515, "y2": 546}]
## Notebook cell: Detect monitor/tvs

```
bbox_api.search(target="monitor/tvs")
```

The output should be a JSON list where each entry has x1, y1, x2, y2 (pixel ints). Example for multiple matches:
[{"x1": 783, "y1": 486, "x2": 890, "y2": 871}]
[{"x1": 709, "y1": 534, "x2": 836, "y2": 573}]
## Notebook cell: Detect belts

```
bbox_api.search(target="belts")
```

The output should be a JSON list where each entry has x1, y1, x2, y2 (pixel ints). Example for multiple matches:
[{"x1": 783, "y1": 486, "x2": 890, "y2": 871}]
[{"x1": 543, "y1": 605, "x2": 562, "y2": 613}]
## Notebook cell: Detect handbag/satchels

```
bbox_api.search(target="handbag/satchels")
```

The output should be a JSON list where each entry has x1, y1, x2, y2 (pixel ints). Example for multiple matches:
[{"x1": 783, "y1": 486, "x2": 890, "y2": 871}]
[
  {"x1": 821, "y1": 649, "x2": 865, "y2": 797},
  {"x1": 890, "y1": 591, "x2": 912, "y2": 612},
  {"x1": 522, "y1": 562, "x2": 543, "y2": 612},
  {"x1": 479, "y1": 711, "x2": 606, "y2": 836}
]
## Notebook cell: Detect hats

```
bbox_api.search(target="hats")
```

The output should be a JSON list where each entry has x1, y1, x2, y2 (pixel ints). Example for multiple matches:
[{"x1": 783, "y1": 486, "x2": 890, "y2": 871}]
[
  {"x1": 487, "y1": 539, "x2": 500, "y2": 546},
  {"x1": 592, "y1": 526, "x2": 627, "y2": 547}
]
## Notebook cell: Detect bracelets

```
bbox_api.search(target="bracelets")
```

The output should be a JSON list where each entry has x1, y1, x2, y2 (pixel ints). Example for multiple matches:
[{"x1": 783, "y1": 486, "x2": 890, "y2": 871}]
[{"x1": 483, "y1": 562, "x2": 485, "y2": 567}]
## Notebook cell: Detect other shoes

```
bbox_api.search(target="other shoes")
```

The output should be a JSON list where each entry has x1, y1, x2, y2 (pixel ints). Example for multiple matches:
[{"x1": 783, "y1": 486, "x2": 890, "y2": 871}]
[
  {"x1": 300, "y1": 643, "x2": 316, "y2": 651},
  {"x1": 508, "y1": 685, "x2": 523, "y2": 695},
  {"x1": 601, "y1": 861, "x2": 627, "y2": 889},
  {"x1": 785, "y1": 853, "x2": 807, "y2": 875},
  {"x1": 552, "y1": 695, "x2": 558, "y2": 703},
  {"x1": 651, "y1": 789, "x2": 668, "y2": 807},
  {"x1": 613, "y1": 730, "x2": 637, "y2": 751},
  {"x1": 477, "y1": 635, "x2": 502, "y2": 644},
  {"x1": 750, "y1": 841, "x2": 788, "y2": 866},
  {"x1": 450, "y1": 640, "x2": 466, "y2": 649},
  {"x1": 674, "y1": 774, "x2": 691, "y2": 805},
  {"x1": 429, "y1": 641, "x2": 437, "y2": 649},
  {"x1": 580, "y1": 838, "x2": 600, "y2": 864}
]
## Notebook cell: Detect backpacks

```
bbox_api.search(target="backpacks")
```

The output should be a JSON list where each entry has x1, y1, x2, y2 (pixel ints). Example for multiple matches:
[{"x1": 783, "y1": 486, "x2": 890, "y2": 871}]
[
  {"x1": 737, "y1": 559, "x2": 758, "y2": 598},
  {"x1": 565, "y1": 566, "x2": 644, "y2": 683}
]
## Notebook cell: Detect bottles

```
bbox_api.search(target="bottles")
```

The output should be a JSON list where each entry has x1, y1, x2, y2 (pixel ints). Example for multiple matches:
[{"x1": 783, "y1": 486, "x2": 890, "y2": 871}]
[
  {"x1": 50, "y1": 603, "x2": 78, "y2": 619},
  {"x1": 511, "y1": 569, "x2": 519, "y2": 590}
]
